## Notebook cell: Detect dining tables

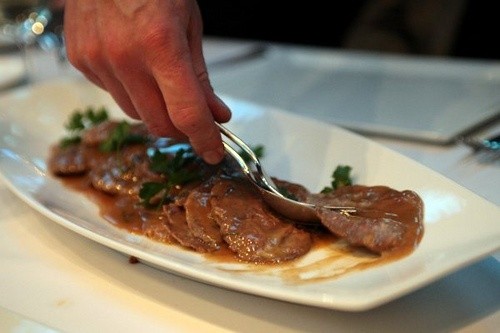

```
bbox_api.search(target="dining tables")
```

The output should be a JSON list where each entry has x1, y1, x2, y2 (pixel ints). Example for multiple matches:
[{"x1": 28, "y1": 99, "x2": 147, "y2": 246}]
[{"x1": 0, "y1": 43, "x2": 499, "y2": 333}]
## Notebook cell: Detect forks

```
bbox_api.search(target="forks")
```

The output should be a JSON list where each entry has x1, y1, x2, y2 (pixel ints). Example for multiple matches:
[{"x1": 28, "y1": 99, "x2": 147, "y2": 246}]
[{"x1": 212, "y1": 120, "x2": 358, "y2": 216}]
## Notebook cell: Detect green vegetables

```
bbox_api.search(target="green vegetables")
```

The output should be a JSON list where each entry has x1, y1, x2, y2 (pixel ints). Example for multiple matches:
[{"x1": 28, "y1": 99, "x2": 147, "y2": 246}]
[{"x1": 59, "y1": 106, "x2": 353, "y2": 212}]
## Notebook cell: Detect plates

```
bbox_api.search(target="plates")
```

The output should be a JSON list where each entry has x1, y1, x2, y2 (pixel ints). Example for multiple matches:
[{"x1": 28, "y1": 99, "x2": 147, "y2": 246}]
[
  {"x1": 200, "y1": 40, "x2": 500, "y2": 151},
  {"x1": 1, "y1": 73, "x2": 500, "y2": 315}
]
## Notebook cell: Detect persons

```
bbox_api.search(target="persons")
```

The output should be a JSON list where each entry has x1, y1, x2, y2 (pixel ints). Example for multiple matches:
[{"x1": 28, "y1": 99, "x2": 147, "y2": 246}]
[{"x1": 61, "y1": 0, "x2": 231, "y2": 165}]
